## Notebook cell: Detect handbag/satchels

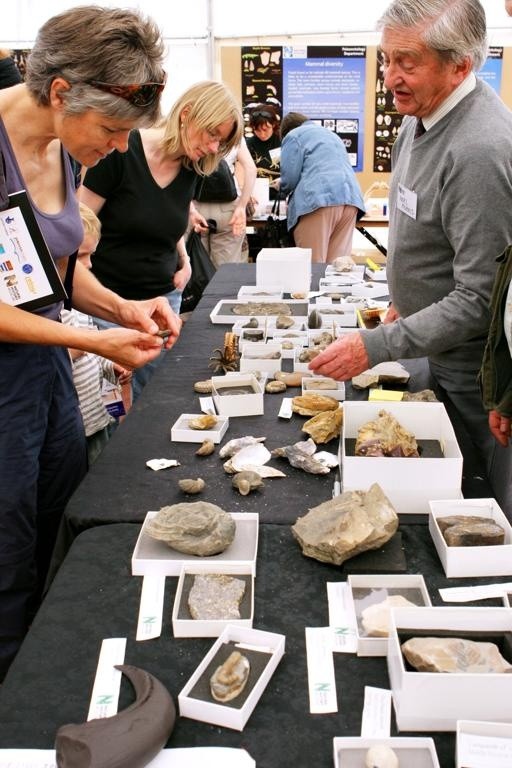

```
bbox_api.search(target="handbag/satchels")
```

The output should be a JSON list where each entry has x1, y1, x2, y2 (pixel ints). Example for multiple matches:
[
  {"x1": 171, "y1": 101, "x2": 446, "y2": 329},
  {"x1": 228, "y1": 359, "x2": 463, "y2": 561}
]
[{"x1": 261, "y1": 185, "x2": 296, "y2": 248}]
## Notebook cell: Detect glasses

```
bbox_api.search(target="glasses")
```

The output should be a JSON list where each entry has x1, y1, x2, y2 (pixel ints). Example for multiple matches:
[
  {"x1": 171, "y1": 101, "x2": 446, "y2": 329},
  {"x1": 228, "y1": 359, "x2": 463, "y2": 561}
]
[
  {"x1": 252, "y1": 111, "x2": 276, "y2": 118},
  {"x1": 84, "y1": 70, "x2": 167, "y2": 108}
]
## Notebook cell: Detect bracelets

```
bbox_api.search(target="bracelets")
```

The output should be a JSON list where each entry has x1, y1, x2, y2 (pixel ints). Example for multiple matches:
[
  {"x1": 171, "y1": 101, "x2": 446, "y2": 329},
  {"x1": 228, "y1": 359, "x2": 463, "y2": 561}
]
[{"x1": 178, "y1": 254, "x2": 190, "y2": 259}]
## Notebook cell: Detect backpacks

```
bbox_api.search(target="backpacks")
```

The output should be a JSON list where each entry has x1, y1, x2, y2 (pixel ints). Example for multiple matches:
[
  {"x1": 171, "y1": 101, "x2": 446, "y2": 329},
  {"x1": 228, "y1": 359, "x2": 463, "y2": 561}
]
[{"x1": 191, "y1": 159, "x2": 237, "y2": 203}]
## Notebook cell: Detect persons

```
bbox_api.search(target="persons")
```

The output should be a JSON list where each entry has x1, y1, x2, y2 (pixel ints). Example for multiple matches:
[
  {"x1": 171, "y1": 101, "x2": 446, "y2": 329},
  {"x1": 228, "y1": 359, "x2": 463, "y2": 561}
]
[
  {"x1": 307, "y1": 0, "x2": 512, "y2": 500},
  {"x1": 75, "y1": 82, "x2": 244, "y2": 406},
  {"x1": 245, "y1": 106, "x2": 282, "y2": 180},
  {"x1": 477, "y1": 244, "x2": 512, "y2": 448},
  {"x1": 60, "y1": 202, "x2": 133, "y2": 467},
  {"x1": 184, "y1": 137, "x2": 258, "y2": 270},
  {"x1": 0, "y1": 6, "x2": 183, "y2": 684},
  {"x1": 272, "y1": 112, "x2": 367, "y2": 264}
]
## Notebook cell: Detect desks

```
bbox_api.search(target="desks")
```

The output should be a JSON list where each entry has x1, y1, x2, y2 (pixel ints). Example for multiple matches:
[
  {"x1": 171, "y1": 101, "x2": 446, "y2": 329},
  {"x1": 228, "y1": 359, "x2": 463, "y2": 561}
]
[
  {"x1": 248, "y1": 161, "x2": 391, "y2": 260},
  {"x1": 0, "y1": 250, "x2": 512, "y2": 767}
]
